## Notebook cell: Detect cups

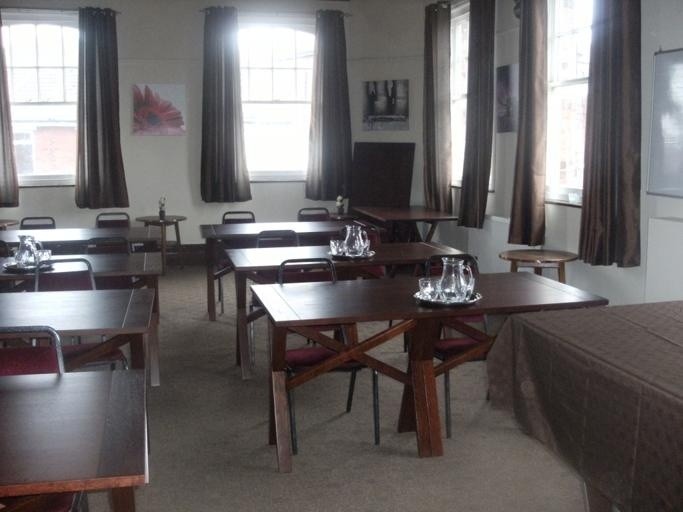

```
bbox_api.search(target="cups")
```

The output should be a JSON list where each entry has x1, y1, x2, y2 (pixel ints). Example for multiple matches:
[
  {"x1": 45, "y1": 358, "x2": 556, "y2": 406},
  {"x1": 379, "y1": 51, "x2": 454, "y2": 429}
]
[
  {"x1": 36, "y1": 249, "x2": 52, "y2": 270},
  {"x1": 419, "y1": 277, "x2": 440, "y2": 299},
  {"x1": 328, "y1": 239, "x2": 345, "y2": 255}
]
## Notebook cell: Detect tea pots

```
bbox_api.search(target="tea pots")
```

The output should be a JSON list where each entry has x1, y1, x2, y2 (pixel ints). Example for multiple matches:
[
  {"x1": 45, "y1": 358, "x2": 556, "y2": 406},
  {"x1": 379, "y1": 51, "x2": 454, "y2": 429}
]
[
  {"x1": 438, "y1": 256, "x2": 473, "y2": 301},
  {"x1": 343, "y1": 224, "x2": 369, "y2": 256},
  {"x1": 15, "y1": 234, "x2": 42, "y2": 268}
]
